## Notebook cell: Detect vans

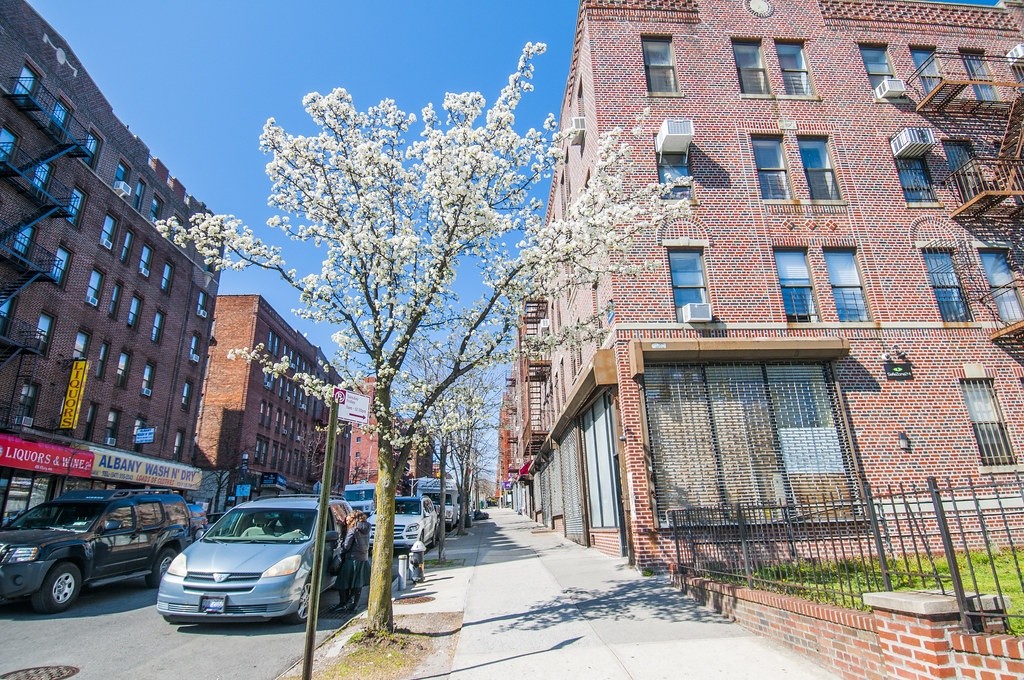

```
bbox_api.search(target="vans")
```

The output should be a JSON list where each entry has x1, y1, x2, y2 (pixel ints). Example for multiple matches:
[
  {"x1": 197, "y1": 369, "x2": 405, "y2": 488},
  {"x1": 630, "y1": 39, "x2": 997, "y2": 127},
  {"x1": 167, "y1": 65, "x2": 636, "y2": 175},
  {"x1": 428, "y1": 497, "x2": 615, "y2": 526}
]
[{"x1": 344, "y1": 482, "x2": 376, "y2": 518}]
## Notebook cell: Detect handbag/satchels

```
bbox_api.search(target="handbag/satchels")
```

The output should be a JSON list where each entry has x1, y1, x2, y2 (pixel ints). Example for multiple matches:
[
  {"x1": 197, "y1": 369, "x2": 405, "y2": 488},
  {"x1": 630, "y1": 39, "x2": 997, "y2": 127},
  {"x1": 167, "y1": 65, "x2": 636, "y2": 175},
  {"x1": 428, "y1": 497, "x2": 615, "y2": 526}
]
[{"x1": 329, "y1": 554, "x2": 343, "y2": 577}]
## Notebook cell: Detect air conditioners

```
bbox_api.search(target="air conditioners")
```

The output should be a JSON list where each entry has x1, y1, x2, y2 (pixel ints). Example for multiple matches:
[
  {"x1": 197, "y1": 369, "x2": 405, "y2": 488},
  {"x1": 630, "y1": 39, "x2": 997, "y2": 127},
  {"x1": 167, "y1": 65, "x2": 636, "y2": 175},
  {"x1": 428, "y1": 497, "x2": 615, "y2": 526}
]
[
  {"x1": 84, "y1": 296, "x2": 98, "y2": 307},
  {"x1": 190, "y1": 354, "x2": 199, "y2": 363},
  {"x1": 196, "y1": 310, "x2": 208, "y2": 319},
  {"x1": 16, "y1": 416, "x2": 33, "y2": 427},
  {"x1": 150, "y1": 216, "x2": 157, "y2": 226},
  {"x1": 540, "y1": 318, "x2": 549, "y2": 332},
  {"x1": 99, "y1": 239, "x2": 112, "y2": 250},
  {"x1": 285, "y1": 396, "x2": 290, "y2": 400},
  {"x1": 892, "y1": 127, "x2": 931, "y2": 156},
  {"x1": 682, "y1": 303, "x2": 713, "y2": 323},
  {"x1": 142, "y1": 387, "x2": 151, "y2": 397},
  {"x1": 264, "y1": 381, "x2": 270, "y2": 387},
  {"x1": 282, "y1": 430, "x2": 287, "y2": 435},
  {"x1": 114, "y1": 180, "x2": 132, "y2": 196},
  {"x1": 876, "y1": 78, "x2": 906, "y2": 97},
  {"x1": 289, "y1": 363, "x2": 296, "y2": 369},
  {"x1": 655, "y1": 119, "x2": 694, "y2": 152},
  {"x1": 1008, "y1": 44, "x2": 1024, "y2": 66},
  {"x1": 296, "y1": 436, "x2": 302, "y2": 441},
  {"x1": 104, "y1": 437, "x2": 116, "y2": 446},
  {"x1": 138, "y1": 267, "x2": 149, "y2": 277},
  {"x1": 299, "y1": 404, "x2": 306, "y2": 410}
]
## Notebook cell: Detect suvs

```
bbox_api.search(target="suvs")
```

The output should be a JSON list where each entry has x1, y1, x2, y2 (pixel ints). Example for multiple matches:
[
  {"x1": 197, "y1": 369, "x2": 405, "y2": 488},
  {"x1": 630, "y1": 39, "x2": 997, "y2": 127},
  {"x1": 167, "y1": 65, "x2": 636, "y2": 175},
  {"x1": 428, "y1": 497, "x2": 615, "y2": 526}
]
[
  {"x1": 365, "y1": 494, "x2": 438, "y2": 556},
  {"x1": 0, "y1": 486, "x2": 194, "y2": 616}
]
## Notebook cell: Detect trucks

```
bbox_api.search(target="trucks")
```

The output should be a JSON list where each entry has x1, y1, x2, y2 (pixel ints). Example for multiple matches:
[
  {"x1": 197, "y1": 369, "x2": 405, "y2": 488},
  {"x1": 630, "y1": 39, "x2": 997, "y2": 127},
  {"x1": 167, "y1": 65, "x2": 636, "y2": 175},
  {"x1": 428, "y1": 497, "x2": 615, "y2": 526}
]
[{"x1": 417, "y1": 475, "x2": 459, "y2": 531}]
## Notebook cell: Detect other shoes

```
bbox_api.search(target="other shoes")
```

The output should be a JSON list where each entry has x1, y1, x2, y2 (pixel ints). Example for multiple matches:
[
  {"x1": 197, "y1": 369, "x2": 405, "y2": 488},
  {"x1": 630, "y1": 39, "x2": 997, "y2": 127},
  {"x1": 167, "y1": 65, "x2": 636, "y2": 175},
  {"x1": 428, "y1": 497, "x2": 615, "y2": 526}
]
[
  {"x1": 334, "y1": 604, "x2": 347, "y2": 613},
  {"x1": 350, "y1": 605, "x2": 357, "y2": 611}
]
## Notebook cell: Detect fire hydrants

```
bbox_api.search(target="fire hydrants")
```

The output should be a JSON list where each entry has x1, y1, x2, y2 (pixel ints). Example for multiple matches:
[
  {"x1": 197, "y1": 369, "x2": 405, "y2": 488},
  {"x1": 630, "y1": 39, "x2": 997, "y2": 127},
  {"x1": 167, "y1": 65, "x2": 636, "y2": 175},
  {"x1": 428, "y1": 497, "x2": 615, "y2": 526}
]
[{"x1": 408, "y1": 539, "x2": 427, "y2": 587}]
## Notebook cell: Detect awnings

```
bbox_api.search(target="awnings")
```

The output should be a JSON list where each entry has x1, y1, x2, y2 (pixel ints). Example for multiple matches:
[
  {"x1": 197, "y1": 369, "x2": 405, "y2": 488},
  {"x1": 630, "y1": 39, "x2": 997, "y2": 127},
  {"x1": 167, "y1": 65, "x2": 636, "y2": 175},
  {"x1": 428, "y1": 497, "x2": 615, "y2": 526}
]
[
  {"x1": 70, "y1": 439, "x2": 202, "y2": 491},
  {"x1": 0, "y1": 434, "x2": 95, "y2": 479},
  {"x1": 518, "y1": 461, "x2": 531, "y2": 482}
]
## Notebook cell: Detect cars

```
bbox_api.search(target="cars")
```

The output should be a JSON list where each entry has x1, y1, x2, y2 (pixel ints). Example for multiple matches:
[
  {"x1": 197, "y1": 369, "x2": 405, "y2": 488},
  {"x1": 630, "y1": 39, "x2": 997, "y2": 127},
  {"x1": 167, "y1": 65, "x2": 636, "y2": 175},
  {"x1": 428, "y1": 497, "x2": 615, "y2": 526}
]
[
  {"x1": 8, "y1": 479, "x2": 49, "y2": 500},
  {"x1": 187, "y1": 502, "x2": 209, "y2": 542},
  {"x1": 0, "y1": 508, "x2": 29, "y2": 529},
  {"x1": 156, "y1": 491, "x2": 356, "y2": 626}
]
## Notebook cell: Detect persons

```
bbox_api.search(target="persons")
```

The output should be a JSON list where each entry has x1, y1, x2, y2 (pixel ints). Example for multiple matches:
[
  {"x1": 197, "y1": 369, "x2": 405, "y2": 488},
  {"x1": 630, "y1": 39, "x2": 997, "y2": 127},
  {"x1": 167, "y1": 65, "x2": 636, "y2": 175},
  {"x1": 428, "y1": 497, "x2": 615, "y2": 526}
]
[{"x1": 330, "y1": 510, "x2": 372, "y2": 613}]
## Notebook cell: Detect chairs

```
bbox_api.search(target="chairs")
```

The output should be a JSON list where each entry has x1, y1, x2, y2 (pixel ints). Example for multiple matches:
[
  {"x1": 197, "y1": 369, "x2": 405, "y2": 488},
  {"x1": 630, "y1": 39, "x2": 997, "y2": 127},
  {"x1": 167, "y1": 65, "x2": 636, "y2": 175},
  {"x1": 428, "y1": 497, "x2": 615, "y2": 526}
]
[{"x1": 247, "y1": 514, "x2": 272, "y2": 536}]
